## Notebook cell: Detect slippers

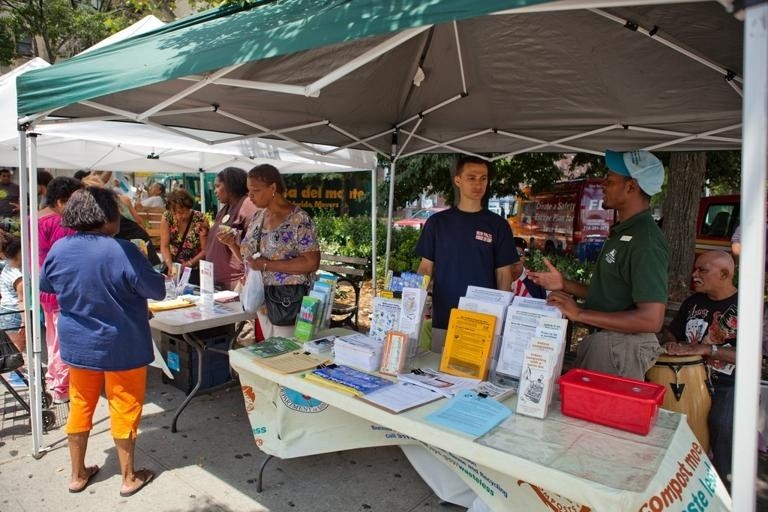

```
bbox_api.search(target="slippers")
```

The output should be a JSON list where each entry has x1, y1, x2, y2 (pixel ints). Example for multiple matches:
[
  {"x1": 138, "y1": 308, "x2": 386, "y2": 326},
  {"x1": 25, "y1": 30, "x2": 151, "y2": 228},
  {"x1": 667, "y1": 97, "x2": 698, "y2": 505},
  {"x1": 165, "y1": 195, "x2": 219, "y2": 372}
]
[
  {"x1": 120, "y1": 468, "x2": 155, "y2": 497},
  {"x1": 69, "y1": 465, "x2": 100, "y2": 493}
]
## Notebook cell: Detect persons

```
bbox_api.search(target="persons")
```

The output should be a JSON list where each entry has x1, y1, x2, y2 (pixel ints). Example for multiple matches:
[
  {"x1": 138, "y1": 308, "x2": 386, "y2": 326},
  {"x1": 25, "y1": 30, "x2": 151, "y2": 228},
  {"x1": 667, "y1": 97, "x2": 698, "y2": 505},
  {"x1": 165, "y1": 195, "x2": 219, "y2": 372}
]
[
  {"x1": 161, "y1": 189, "x2": 210, "y2": 285},
  {"x1": 0, "y1": 169, "x2": 166, "y2": 404},
  {"x1": 39, "y1": 186, "x2": 166, "y2": 497},
  {"x1": 196, "y1": 167, "x2": 261, "y2": 347},
  {"x1": 510, "y1": 237, "x2": 547, "y2": 298},
  {"x1": 730, "y1": 226, "x2": 741, "y2": 256},
  {"x1": 663, "y1": 250, "x2": 739, "y2": 494},
  {"x1": 416, "y1": 155, "x2": 518, "y2": 353},
  {"x1": 216, "y1": 164, "x2": 322, "y2": 340},
  {"x1": 525, "y1": 149, "x2": 672, "y2": 381}
]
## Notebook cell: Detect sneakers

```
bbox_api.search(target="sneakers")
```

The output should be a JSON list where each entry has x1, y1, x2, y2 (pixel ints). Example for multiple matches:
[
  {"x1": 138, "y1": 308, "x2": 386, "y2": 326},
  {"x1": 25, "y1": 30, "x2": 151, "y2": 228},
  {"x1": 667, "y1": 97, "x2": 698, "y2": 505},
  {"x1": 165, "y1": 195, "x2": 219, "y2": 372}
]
[{"x1": 52, "y1": 398, "x2": 69, "y2": 404}]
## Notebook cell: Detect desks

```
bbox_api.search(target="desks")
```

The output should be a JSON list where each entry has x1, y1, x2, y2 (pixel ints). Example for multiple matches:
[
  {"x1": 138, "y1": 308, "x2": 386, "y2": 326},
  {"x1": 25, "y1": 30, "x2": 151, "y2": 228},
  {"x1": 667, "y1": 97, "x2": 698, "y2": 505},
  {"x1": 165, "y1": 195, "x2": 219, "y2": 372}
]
[
  {"x1": 136, "y1": 280, "x2": 257, "y2": 434},
  {"x1": 227, "y1": 328, "x2": 731, "y2": 512}
]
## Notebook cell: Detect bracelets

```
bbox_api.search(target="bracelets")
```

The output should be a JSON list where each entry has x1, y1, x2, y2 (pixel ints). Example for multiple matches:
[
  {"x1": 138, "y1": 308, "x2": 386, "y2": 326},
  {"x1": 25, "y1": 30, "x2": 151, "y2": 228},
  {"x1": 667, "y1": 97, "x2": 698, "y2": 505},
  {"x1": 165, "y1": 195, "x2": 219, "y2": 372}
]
[
  {"x1": 263, "y1": 259, "x2": 268, "y2": 272},
  {"x1": 187, "y1": 261, "x2": 194, "y2": 266}
]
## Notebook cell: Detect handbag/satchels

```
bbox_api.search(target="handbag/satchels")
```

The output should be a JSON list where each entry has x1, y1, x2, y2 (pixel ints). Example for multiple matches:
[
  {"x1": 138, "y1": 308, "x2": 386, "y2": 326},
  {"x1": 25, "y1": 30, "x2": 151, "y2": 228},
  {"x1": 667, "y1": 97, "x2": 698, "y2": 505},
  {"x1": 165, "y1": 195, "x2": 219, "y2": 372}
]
[
  {"x1": 262, "y1": 283, "x2": 309, "y2": 326},
  {"x1": 159, "y1": 254, "x2": 175, "y2": 273}
]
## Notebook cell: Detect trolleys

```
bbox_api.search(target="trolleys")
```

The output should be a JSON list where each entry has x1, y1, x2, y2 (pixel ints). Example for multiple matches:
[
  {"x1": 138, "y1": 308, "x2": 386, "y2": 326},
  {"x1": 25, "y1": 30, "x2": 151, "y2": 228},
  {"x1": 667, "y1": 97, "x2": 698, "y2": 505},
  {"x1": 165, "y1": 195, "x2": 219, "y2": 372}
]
[{"x1": 0, "y1": 303, "x2": 56, "y2": 444}]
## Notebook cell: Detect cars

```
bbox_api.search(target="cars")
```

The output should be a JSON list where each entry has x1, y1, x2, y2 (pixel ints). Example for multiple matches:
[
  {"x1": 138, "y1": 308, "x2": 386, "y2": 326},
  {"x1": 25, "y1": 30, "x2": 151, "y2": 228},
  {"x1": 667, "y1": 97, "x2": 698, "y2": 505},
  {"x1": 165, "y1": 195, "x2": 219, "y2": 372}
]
[
  {"x1": 651, "y1": 214, "x2": 661, "y2": 222},
  {"x1": 392, "y1": 207, "x2": 449, "y2": 232}
]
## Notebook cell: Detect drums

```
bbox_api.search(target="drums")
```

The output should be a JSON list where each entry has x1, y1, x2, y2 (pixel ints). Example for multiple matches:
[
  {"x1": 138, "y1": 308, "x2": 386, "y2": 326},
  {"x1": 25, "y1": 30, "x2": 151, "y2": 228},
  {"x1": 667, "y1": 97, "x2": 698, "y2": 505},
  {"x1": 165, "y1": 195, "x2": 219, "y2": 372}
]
[{"x1": 644, "y1": 354, "x2": 712, "y2": 454}]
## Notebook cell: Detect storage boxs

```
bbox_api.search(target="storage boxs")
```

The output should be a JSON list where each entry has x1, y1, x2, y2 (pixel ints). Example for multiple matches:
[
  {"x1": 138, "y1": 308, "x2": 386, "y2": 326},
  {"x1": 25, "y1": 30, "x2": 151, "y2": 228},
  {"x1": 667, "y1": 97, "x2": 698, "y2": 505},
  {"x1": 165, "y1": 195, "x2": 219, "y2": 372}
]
[{"x1": 158, "y1": 330, "x2": 227, "y2": 397}]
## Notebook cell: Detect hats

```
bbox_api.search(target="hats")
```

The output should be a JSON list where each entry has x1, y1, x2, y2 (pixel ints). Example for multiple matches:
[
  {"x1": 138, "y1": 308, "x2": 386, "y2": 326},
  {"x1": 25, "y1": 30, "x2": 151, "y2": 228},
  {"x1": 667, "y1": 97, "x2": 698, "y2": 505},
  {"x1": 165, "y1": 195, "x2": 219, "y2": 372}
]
[{"x1": 604, "y1": 148, "x2": 664, "y2": 197}]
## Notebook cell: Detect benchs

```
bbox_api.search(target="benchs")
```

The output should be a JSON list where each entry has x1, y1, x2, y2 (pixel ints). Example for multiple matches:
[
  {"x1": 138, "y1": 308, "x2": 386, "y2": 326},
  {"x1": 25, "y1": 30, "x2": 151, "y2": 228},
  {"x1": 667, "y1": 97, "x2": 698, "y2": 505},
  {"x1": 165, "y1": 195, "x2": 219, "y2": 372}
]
[{"x1": 314, "y1": 253, "x2": 367, "y2": 331}]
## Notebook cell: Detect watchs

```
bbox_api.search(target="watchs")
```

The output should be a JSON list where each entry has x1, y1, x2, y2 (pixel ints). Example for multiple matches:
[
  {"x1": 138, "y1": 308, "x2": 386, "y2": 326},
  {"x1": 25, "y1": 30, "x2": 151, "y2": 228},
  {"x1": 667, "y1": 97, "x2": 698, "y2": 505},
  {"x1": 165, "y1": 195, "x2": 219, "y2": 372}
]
[{"x1": 711, "y1": 344, "x2": 717, "y2": 357}]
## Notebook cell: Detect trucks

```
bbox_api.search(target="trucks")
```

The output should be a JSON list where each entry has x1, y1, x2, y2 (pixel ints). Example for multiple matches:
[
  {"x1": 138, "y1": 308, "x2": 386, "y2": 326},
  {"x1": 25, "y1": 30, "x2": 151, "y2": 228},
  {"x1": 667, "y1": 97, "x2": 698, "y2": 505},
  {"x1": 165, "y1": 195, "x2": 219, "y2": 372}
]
[
  {"x1": 507, "y1": 177, "x2": 620, "y2": 262},
  {"x1": 488, "y1": 198, "x2": 512, "y2": 218},
  {"x1": 146, "y1": 172, "x2": 376, "y2": 217},
  {"x1": 691, "y1": 195, "x2": 742, "y2": 295}
]
